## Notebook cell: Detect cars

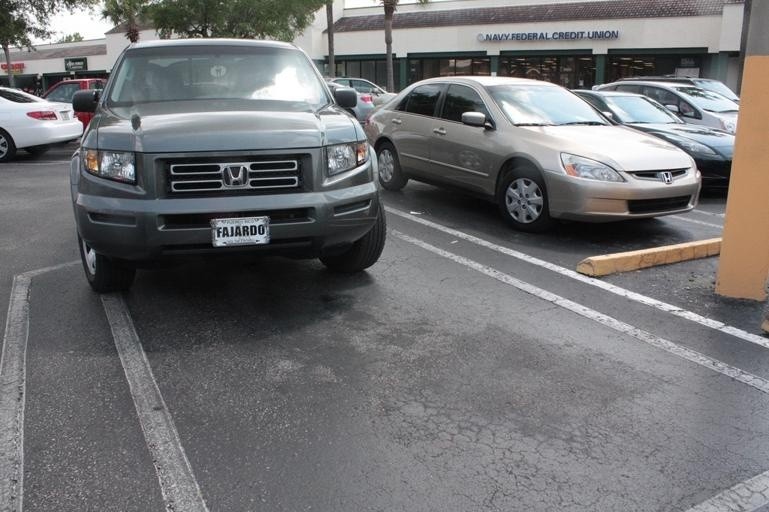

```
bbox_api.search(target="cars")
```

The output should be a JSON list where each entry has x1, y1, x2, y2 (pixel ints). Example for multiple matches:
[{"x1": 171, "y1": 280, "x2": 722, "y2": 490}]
[
  {"x1": 322, "y1": 79, "x2": 375, "y2": 124},
  {"x1": 324, "y1": 77, "x2": 398, "y2": 125},
  {"x1": 40, "y1": 77, "x2": 107, "y2": 130},
  {"x1": 569, "y1": 76, "x2": 740, "y2": 190},
  {"x1": 364, "y1": 76, "x2": 704, "y2": 233},
  {"x1": 0, "y1": 86, "x2": 85, "y2": 160}
]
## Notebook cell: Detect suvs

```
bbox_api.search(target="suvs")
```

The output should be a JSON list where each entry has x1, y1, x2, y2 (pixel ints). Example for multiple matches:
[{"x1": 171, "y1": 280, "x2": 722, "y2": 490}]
[{"x1": 68, "y1": 37, "x2": 388, "y2": 294}]
